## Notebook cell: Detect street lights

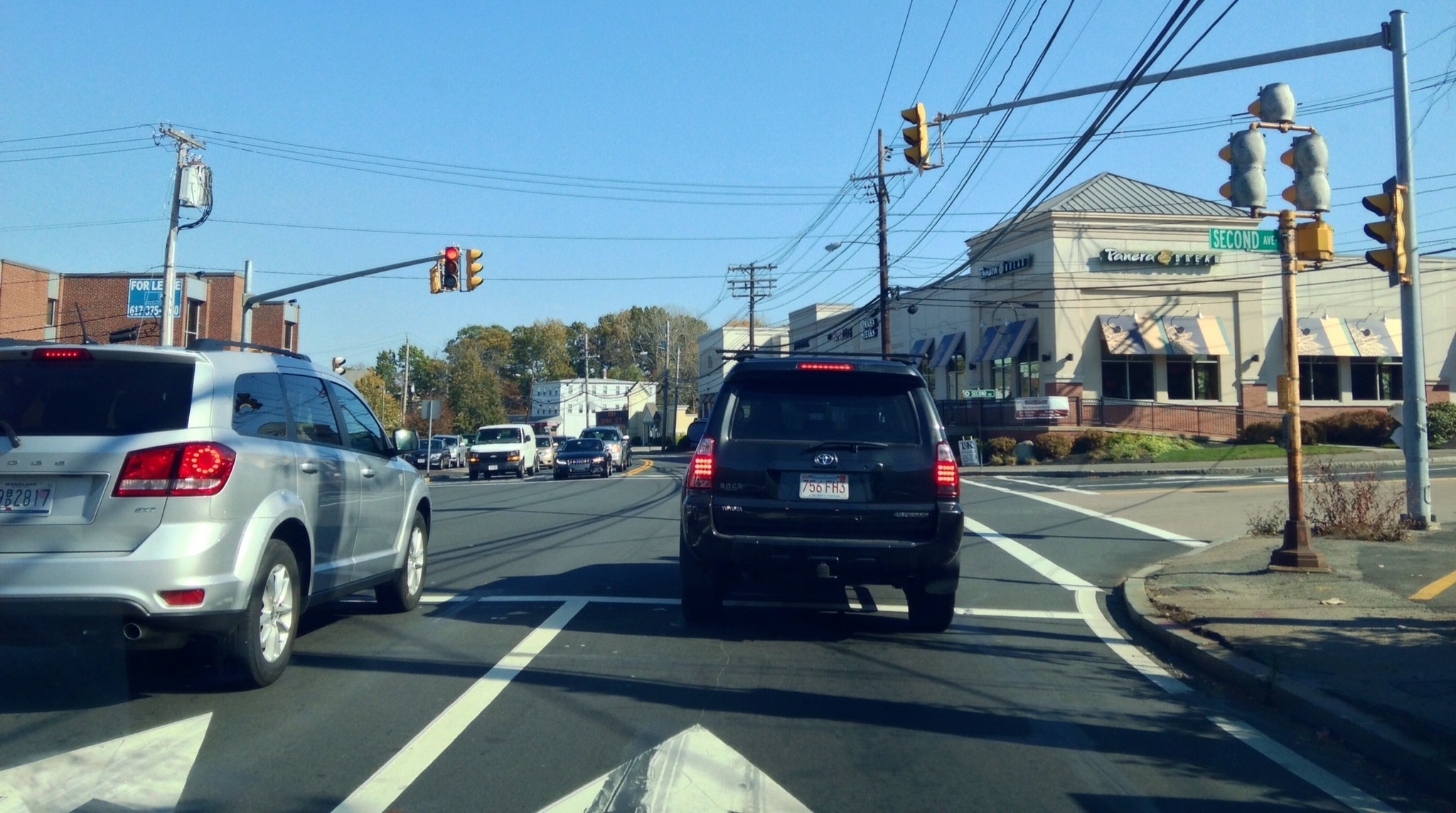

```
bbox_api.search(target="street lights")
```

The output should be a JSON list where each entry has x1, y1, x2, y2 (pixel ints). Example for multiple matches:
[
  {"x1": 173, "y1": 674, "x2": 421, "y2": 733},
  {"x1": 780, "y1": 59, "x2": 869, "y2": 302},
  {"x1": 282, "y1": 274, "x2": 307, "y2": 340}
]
[
  {"x1": 640, "y1": 351, "x2": 669, "y2": 452},
  {"x1": 824, "y1": 240, "x2": 891, "y2": 362},
  {"x1": 514, "y1": 373, "x2": 534, "y2": 424},
  {"x1": 376, "y1": 385, "x2": 385, "y2": 429},
  {"x1": 445, "y1": 247, "x2": 458, "y2": 288},
  {"x1": 334, "y1": 357, "x2": 346, "y2": 375}
]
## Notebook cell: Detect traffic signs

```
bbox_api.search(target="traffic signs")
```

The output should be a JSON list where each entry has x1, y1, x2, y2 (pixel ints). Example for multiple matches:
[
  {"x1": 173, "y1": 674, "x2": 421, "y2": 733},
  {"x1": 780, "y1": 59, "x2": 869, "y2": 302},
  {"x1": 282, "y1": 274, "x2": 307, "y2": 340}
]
[
  {"x1": 963, "y1": 389, "x2": 995, "y2": 397},
  {"x1": 1210, "y1": 227, "x2": 1283, "y2": 252}
]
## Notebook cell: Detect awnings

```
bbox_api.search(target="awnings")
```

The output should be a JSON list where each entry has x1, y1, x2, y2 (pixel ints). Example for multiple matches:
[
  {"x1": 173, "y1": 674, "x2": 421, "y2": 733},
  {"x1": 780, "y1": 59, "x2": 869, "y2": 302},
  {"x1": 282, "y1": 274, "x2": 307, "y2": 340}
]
[
  {"x1": 969, "y1": 317, "x2": 1038, "y2": 364},
  {"x1": 1284, "y1": 315, "x2": 1404, "y2": 355},
  {"x1": 926, "y1": 331, "x2": 965, "y2": 370},
  {"x1": 1098, "y1": 313, "x2": 1230, "y2": 354},
  {"x1": 908, "y1": 338, "x2": 935, "y2": 370}
]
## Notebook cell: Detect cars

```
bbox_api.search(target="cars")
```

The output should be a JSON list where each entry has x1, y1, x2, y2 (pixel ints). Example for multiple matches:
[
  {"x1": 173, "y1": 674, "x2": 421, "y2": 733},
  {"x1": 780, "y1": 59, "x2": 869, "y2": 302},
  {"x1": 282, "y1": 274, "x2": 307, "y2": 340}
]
[
  {"x1": 551, "y1": 435, "x2": 568, "y2": 453},
  {"x1": 404, "y1": 439, "x2": 451, "y2": 470},
  {"x1": 625, "y1": 440, "x2": 633, "y2": 466},
  {"x1": 461, "y1": 433, "x2": 477, "y2": 452},
  {"x1": 553, "y1": 439, "x2": 613, "y2": 478},
  {"x1": 534, "y1": 435, "x2": 558, "y2": 469}
]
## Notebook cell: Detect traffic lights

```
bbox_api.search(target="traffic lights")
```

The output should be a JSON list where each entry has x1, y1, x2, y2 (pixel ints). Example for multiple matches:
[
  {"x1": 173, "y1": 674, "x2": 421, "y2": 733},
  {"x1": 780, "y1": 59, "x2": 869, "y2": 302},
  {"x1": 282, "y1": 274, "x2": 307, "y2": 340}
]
[
  {"x1": 1363, "y1": 191, "x2": 1395, "y2": 271},
  {"x1": 471, "y1": 249, "x2": 484, "y2": 288},
  {"x1": 901, "y1": 106, "x2": 920, "y2": 168}
]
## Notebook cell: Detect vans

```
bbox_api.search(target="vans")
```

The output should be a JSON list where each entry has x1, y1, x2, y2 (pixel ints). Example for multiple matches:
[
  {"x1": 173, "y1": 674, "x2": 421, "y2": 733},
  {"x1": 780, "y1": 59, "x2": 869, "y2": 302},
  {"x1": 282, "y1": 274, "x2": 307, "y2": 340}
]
[{"x1": 469, "y1": 423, "x2": 538, "y2": 480}]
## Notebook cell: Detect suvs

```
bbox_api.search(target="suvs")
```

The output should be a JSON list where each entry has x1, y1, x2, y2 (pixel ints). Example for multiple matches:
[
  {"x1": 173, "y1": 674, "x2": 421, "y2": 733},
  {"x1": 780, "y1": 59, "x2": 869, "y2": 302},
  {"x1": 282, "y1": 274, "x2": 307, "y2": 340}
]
[
  {"x1": 679, "y1": 356, "x2": 964, "y2": 634},
  {"x1": 431, "y1": 433, "x2": 466, "y2": 468},
  {"x1": 578, "y1": 426, "x2": 630, "y2": 472},
  {"x1": 1, "y1": 301, "x2": 431, "y2": 687}
]
